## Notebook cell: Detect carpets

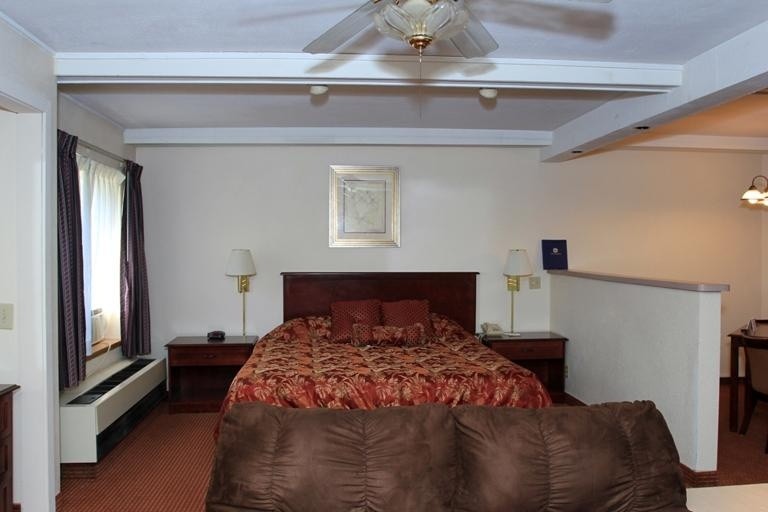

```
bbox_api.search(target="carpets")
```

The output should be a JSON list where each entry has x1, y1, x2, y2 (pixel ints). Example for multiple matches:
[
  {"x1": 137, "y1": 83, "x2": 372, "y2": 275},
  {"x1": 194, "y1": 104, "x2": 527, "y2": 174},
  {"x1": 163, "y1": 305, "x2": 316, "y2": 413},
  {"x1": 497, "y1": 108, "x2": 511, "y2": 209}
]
[
  {"x1": 56, "y1": 402, "x2": 221, "y2": 512},
  {"x1": 717, "y1": 382, "x2": 768, "y2": 487}
]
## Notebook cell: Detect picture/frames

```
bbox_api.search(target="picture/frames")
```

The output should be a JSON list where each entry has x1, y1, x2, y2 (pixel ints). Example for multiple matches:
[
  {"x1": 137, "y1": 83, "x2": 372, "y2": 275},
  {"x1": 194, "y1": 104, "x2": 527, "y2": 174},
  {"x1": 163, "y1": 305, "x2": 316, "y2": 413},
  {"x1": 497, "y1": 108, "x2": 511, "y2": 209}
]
[{"x1": 328, "y1": 165, "x2": 399, "y2": 248}]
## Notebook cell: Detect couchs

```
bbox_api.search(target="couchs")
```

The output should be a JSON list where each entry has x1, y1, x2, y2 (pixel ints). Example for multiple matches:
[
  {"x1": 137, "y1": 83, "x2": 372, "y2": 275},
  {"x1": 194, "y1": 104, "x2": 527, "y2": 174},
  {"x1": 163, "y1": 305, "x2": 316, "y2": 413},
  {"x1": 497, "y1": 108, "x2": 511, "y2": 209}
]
[{"x1": 204, "y1": 400, "x2": 689, "y2": 512}]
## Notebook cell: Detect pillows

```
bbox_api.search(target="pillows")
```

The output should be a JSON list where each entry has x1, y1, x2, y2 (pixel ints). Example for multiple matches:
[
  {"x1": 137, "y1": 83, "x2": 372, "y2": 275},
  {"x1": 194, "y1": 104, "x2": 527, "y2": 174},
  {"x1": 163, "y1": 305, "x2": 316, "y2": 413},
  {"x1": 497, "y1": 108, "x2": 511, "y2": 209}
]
[
  {"x1": 328, "y1": 299, "x2": 380, "y2": 344},
  {"x1": 306, "y1": 312, "x2": 466, "y2": 341},
  {"x1": 350, "y1": 323, "x2": 425, "y2": 346},
  {"x1": 380, "y1": 299, "x2": 435, "y2": 344}
]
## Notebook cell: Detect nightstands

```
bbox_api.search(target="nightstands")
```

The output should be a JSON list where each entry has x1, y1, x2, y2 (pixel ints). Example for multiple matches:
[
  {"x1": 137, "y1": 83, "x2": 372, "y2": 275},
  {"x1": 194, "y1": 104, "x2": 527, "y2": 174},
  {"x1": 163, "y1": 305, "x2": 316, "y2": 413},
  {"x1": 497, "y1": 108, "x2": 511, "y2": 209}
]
[
  {"x1": 475, "y1": 332, "x2": 567, "y2": 393},
  {"x1": 164, "y1": 336, "x2": 259, "y2": 415}
]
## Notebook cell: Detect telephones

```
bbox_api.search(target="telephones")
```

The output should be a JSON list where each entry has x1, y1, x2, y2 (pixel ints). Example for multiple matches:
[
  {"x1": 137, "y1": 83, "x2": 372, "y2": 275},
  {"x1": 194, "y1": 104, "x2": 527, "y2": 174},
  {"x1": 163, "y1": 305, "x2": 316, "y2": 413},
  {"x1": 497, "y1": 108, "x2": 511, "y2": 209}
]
[{"x1": 481, "y1": 322, "x2": 504, "y2": 336}]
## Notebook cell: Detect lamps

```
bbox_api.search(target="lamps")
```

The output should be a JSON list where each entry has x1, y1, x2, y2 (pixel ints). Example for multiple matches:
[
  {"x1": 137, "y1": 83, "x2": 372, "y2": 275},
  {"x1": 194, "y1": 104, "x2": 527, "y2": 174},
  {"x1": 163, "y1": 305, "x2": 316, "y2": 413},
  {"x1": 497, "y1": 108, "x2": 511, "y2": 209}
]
[
  {"x1": 224, "y1": 249, "x2": 256, "y2": 337},
  {"x1": 502, "y1": 249, "x2": 532, "y2": 333},
  {"x1": 739, "y1": 175, "x2": 767, "y2": 206},
  {"x1": 376, "y1": 0, "x2": 468, "y2": 79}
]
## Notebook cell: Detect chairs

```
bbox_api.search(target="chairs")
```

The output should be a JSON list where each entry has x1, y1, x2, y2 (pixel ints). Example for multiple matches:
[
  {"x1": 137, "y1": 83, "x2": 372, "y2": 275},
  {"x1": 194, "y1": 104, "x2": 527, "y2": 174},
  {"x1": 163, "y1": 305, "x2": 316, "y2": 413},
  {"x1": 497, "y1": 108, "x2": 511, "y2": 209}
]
[{"x1": 737, "y1": 337, "x2": 767, "y2": 455}]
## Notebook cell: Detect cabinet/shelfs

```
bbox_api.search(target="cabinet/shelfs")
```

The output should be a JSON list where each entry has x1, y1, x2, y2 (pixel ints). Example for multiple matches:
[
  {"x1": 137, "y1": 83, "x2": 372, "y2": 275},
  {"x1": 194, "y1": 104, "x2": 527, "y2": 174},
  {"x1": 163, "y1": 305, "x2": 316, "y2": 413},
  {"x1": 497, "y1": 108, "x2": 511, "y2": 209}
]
[
  {"x1": 0, "y1": 384, "x2": 21, "y2": 512},
  {"x1": 59, "y1": 356, "x2": 167, "y2": 463}
]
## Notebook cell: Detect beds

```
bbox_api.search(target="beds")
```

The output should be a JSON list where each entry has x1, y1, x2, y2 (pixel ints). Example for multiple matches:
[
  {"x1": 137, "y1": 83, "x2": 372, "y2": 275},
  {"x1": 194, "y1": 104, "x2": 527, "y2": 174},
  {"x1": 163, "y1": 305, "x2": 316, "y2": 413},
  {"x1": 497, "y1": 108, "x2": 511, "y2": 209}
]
[{"x1": 213, "y1": 271, "x2": 552, "y2": 442}]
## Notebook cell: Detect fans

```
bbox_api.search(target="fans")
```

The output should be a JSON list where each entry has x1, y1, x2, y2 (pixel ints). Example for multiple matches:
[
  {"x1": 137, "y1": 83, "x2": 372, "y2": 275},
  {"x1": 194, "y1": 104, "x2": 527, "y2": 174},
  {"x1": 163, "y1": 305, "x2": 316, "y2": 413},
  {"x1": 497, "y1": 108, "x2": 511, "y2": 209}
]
[{"x1": 302, "y1": 0, "x2": 498, "y2": 59}]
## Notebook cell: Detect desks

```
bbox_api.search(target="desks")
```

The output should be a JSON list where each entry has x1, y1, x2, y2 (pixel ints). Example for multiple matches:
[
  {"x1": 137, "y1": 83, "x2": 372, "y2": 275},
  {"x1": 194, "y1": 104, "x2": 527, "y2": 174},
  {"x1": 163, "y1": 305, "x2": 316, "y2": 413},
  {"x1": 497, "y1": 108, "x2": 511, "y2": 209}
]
[{"x1": 726, "y1": 323, "x2": 767, "y2": 433}]
